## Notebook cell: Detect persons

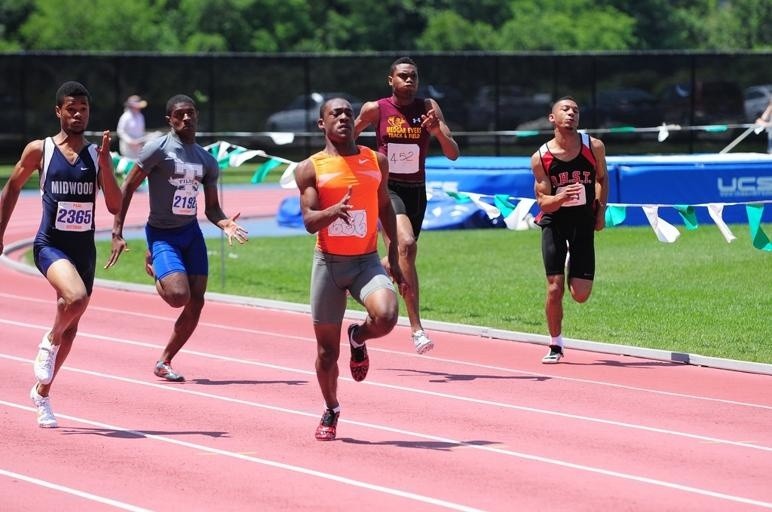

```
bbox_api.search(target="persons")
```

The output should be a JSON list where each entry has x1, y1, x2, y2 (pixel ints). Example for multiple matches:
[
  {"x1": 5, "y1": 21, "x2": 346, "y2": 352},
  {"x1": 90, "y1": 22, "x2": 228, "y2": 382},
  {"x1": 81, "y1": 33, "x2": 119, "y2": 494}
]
[
  {"x1": 117, "y1": 94, "x2": 149, "y2": 159},
  {"x1": 358, "y1": 56, "x2": 458, "y2": 353},
  {"x1": 294, "y1": 98, "x2": 413, "y2": 439},
  {"x1": 103, "y1": 94, "x2": 252, "y2": 381},
  {"x1": 533, "y1": 96, "x2": 609, "y2": 365},
  {"x1": 761, "y1": 97, "x2": 771, "y2": 154}
]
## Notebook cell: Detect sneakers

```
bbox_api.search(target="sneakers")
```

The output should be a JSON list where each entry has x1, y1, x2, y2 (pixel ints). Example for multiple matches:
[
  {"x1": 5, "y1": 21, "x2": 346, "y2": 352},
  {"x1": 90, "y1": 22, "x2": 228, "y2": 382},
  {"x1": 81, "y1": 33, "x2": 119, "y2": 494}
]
[
  {"x1": 34, "y1": 330, "x2": 60, "y2": 384},
  {"x1": 145, "y1": 250, "x2": 152, "y2": 276},
  {"x1": 315, "y1": 409, "x2": 340, "y2": 441},
  {"x1": 412, "y1": 330, "x2": 432, "y2": 354},
  {"x1": 154, "y1": 360, "x2": 183, "y2": 381},
  {"x1": 348, "y1": 323, "x2": 369, "y2": 381},
  {"x1": 542, "y1": 345, "x2": 564, "y2": 364},
  {"x1": 30, "y1": 381, "x2": 57, "y2": 427}
]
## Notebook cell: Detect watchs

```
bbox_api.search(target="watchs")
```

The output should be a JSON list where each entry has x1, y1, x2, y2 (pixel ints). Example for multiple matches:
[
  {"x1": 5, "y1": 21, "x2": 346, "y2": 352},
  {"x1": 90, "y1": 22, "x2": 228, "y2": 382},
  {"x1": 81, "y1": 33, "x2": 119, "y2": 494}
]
[{"x1": 1, "y1": 81, "x2": 125, "y2": 428}]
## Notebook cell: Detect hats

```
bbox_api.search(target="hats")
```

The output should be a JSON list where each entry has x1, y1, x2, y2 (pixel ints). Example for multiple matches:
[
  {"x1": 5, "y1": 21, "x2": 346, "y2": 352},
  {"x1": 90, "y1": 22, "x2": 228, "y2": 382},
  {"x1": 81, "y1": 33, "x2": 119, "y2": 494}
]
[{"x1": 124, "y1": 95, "x2": 147, "y2": 108}]
